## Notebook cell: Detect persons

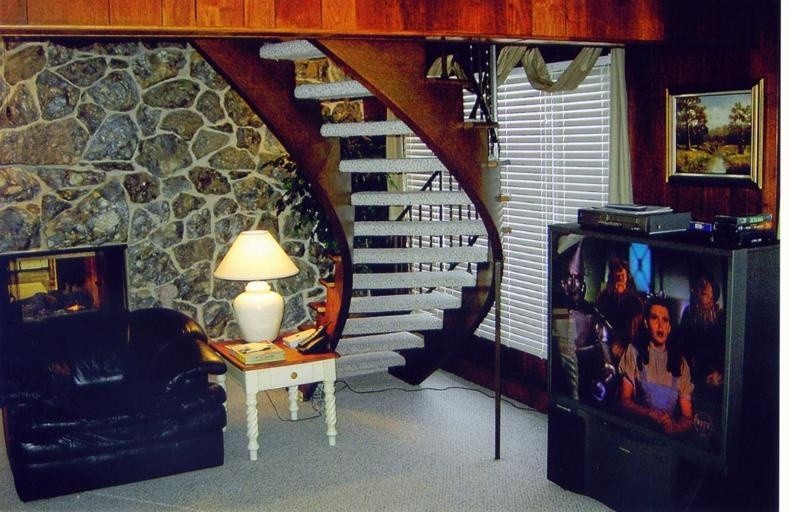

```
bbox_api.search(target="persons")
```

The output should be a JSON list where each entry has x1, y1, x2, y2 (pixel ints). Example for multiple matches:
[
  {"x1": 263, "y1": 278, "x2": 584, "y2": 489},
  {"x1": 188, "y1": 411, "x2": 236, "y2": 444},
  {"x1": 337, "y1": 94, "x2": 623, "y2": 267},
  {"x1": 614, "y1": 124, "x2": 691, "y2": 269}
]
[{"x1": 554, "y1": 240, "x2": 723, "y2": 439}]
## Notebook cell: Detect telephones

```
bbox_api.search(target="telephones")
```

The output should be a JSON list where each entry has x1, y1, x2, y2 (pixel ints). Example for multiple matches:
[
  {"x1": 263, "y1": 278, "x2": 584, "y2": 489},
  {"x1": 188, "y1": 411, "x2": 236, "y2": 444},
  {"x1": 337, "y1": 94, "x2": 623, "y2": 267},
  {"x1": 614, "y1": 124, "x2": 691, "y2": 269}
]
[{"x1": 295, "y1": 320, "x2": 332, "y2": 355}]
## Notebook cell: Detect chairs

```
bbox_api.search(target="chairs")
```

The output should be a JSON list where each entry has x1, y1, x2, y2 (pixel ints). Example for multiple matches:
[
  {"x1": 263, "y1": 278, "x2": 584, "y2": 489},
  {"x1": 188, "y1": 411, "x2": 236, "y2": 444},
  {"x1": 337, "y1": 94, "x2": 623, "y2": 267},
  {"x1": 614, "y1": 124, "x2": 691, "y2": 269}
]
[{"x1": 0, "y1": 307, "x2": 231, "y2": 502}]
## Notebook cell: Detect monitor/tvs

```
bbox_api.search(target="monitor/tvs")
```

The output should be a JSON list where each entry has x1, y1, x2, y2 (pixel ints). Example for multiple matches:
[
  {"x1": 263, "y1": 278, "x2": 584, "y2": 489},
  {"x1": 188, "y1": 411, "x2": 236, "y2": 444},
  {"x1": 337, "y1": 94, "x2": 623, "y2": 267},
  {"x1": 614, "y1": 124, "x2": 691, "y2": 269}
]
[{"x1": 547, "y1": 222, "x2": 780, "y2": 473}]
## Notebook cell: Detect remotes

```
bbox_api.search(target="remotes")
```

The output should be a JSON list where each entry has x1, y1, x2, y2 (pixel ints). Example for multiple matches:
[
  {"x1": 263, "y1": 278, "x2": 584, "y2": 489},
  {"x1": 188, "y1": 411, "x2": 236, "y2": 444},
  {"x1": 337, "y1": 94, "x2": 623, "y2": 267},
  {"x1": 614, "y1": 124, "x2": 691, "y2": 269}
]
[{"x1": 605, "y1": 204, "x2": 647, "y2": 212}]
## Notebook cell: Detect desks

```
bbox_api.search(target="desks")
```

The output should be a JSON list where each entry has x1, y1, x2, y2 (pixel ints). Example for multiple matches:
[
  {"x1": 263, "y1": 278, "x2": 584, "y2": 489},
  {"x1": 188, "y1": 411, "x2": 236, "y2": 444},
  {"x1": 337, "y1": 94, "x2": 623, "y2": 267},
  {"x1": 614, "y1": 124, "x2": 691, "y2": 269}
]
[{"x1": 203, "y1": 330, "x2": 342, "y2": 463}]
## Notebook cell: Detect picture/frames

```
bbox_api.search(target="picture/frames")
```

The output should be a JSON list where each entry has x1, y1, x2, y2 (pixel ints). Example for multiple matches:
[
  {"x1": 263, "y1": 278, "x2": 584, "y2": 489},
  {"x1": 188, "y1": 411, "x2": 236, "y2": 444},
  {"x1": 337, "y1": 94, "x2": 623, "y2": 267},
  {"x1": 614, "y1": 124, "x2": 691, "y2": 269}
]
[{"x1": 661, "y1": 76, "x2": 769, "y2": 194}]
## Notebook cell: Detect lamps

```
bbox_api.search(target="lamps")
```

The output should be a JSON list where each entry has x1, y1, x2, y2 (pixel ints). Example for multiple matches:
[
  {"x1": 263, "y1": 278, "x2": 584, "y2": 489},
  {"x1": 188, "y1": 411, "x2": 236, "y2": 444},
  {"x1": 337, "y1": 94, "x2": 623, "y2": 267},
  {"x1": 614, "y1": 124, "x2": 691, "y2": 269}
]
[{"x1": 208, "y1": 229, "x2": 303, "y2": 346}]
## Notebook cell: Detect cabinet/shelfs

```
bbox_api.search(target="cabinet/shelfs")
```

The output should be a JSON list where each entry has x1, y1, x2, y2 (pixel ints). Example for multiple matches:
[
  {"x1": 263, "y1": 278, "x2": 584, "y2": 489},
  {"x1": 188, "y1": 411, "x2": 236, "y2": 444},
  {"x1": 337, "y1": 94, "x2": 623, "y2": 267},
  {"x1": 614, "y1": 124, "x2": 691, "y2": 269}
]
[{"x1": 538, "y1": 214, "x2": 779, "y2": 509}]
